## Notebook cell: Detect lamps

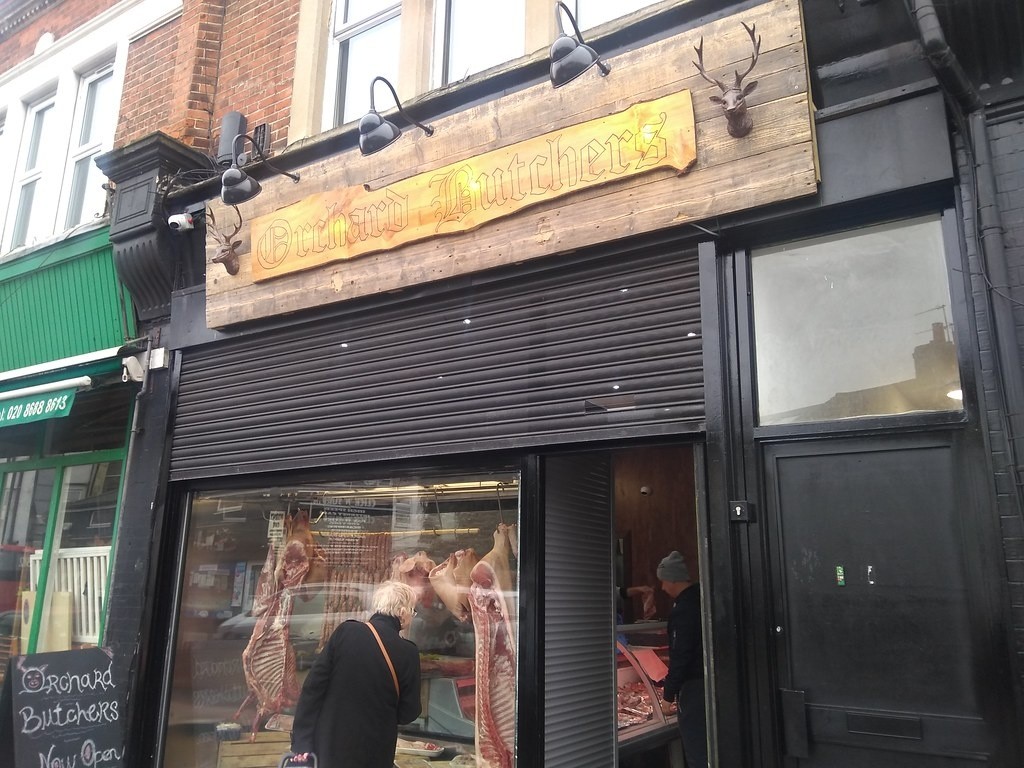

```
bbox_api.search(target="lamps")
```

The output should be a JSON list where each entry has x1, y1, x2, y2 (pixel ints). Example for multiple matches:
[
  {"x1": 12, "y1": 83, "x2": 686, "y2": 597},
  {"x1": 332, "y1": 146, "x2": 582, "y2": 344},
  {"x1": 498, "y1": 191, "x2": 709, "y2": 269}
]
[
  {"x1": 357, "y1": 75, "x2": 435, "y2": 157},
  {"x1": 221, "y1": 133, "x2": 300, "y2": 205},
  {"x1": 550, "y1": 1, "x2": 611, "y2": 90}
]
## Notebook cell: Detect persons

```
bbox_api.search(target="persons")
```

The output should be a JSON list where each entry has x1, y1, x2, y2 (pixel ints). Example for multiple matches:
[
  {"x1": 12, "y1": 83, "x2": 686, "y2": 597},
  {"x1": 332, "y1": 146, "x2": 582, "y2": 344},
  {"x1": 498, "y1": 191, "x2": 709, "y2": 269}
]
[
  {"x1": 615, "y1": 585, "x2": 654, "y2": 601},
  {"x1": 656, "y1": 550, "x2": 709, "y2": 768},
  {"x1": 289, "y1": 580, "x2": 422, "y2": 768}
]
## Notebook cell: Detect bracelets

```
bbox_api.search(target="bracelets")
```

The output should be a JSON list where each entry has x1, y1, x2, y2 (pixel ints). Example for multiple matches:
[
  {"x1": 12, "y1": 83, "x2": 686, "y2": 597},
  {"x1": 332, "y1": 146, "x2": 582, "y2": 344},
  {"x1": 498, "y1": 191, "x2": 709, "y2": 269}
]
[{"x1": 620, "y1": 587, "x2": 629, "y2": 599}]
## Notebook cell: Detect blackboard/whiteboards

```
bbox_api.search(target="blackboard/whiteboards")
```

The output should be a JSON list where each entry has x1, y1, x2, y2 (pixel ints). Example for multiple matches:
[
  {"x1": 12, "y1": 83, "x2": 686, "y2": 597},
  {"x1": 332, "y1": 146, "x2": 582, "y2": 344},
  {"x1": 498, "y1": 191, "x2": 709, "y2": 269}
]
[{"x1": 9, "y1": 647, "x2": 127, "y2": 768}]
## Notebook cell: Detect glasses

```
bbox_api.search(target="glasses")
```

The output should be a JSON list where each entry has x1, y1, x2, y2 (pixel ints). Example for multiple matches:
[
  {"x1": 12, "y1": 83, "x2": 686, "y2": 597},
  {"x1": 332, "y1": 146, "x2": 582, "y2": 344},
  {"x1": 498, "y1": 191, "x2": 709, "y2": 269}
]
[{"x1": 411, "y1": 608, "x2": 418, "y2": 618}]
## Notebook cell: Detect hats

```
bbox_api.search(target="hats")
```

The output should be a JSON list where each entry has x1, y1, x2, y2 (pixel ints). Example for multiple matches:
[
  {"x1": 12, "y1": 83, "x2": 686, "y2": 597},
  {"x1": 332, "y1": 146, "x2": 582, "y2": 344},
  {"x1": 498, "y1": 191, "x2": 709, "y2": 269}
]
[{"x1": 656, "y1": 550, "x2": 695, "y2": 581}]
